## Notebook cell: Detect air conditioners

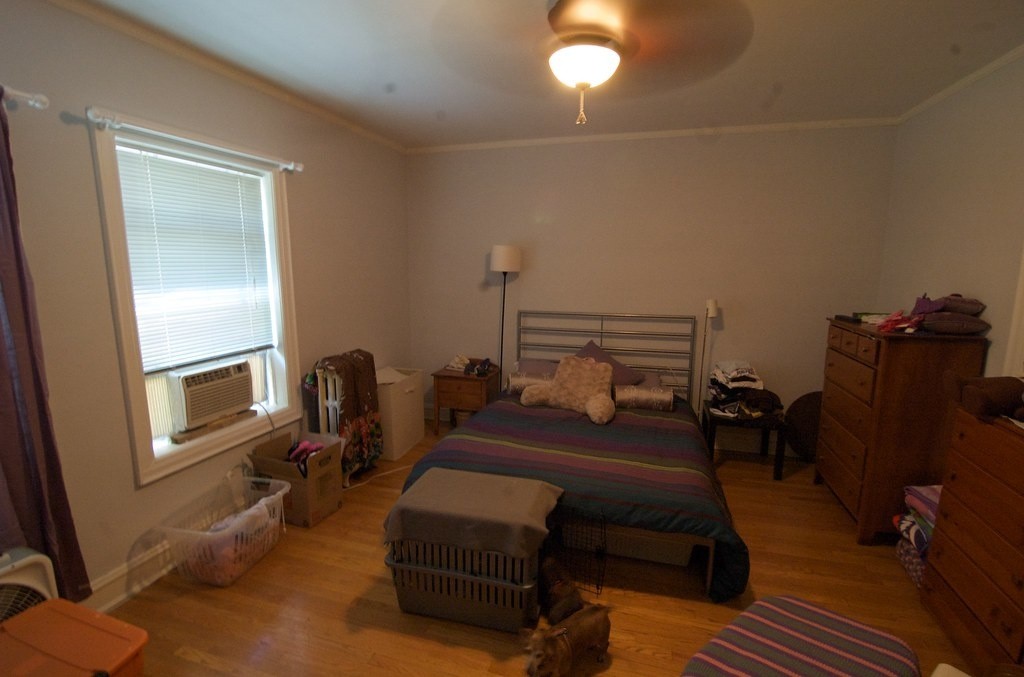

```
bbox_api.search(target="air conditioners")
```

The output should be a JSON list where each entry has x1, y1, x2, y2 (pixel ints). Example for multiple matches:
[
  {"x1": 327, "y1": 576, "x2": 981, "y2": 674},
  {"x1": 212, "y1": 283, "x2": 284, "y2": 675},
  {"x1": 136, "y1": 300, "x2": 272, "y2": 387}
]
[{"x1": 166, "y1": 358, "x2": 253, "y2": 434}]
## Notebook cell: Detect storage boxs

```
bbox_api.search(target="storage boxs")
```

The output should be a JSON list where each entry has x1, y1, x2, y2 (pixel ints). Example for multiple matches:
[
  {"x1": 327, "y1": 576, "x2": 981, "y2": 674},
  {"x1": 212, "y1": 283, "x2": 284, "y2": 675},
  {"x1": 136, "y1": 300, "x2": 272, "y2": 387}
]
[
  {"x1": 159, "y1": 478, "x2": 290, "y2": 587},
  {"x1": 0, "y1": 598, "x2": 149, "y2": 677},
  {"x1": 252, "y1": 430, "x2": 347, "y2": 529}
]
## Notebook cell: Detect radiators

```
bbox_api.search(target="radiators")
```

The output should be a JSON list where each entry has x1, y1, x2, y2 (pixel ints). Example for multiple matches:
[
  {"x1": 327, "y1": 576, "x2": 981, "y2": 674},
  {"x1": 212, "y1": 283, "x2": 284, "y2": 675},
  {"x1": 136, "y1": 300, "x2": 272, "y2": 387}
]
[{"x1": 316, "y1": 366, "x2": 344, "y2": 436}]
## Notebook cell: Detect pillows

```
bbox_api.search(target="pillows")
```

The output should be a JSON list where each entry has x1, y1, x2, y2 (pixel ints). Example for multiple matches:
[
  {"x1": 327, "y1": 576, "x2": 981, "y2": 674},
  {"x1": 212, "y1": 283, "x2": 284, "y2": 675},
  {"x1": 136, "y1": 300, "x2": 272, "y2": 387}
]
[{"x1": 525, "y1": 339, "x2": 674, "y2": 411}]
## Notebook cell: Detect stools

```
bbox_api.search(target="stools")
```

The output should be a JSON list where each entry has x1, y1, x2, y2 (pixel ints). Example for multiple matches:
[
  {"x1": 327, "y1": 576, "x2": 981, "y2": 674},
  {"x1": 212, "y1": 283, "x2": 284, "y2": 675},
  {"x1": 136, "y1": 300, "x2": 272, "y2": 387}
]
[{"x1": 688, "y1": 595, "x2": 917, "y2": 677}]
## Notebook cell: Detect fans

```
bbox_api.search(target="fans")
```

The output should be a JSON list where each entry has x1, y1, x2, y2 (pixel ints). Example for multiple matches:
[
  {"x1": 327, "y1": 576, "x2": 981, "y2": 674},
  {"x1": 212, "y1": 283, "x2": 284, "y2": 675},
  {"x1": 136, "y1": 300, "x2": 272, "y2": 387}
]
[{"x1": 0, "y1": 547, "x2": 58, "y2": 619}]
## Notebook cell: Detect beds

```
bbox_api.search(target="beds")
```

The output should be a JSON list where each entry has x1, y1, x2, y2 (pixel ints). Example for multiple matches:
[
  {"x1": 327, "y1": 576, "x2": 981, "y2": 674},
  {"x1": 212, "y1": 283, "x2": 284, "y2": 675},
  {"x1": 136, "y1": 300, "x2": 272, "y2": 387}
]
[{"x1": 403, "y1": 308, "x2": 750, "y2": 605}]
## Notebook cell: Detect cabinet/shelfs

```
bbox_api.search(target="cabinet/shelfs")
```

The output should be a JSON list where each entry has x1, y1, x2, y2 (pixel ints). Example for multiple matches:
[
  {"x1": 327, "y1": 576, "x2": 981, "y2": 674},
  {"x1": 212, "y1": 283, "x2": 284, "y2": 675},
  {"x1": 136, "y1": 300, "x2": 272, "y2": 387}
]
[
  {"x1": 813, "y1": 316, "x2": 988, "y2": 544},
  {"x1": 917, "y1": 405, "x2": 1024, "y2": 677}
]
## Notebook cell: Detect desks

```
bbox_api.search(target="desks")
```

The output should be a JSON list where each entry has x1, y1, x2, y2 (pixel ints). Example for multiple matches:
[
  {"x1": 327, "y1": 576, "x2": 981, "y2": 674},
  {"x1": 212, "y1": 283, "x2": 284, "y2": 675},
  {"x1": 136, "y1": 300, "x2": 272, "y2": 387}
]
[{"x1": 702, "y1": 401, "x2": 788, "y2": 481}]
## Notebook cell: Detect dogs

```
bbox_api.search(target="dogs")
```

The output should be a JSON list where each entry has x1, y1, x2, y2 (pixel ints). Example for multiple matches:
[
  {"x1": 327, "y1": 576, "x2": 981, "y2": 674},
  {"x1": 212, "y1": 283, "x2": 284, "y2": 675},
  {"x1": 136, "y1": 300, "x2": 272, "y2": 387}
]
[{"x1": 519, "y1": 603, "x2": 611, "y2": 677}]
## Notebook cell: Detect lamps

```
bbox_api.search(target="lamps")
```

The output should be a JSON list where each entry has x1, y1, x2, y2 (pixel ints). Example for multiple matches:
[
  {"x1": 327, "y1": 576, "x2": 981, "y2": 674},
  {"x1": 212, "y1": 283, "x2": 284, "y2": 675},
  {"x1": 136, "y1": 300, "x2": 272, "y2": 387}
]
[
  {"x1": 547, "y1": 28, "x2": 622, "y2": 124},
  {"x1": 490, "y1": 245, "x2": 521, "y2": 394},
  {"x1": 697, "y1": 299, "x2": 718, "y2": 419}
]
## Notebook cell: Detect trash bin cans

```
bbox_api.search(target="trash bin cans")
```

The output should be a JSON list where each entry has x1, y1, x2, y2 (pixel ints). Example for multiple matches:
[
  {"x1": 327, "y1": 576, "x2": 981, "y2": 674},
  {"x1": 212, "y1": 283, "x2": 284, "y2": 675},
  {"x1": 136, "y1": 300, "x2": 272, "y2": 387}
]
[{"x1": 453, "y1": 409, "x2": 472, "y2": 427}]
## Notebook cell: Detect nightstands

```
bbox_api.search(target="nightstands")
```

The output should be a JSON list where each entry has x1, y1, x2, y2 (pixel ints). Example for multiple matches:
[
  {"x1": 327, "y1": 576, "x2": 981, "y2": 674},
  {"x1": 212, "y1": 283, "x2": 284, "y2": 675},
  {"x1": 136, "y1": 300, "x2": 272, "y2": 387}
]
[{"x1": 431, "y1": 365, "x2": 499, "y2": 435}]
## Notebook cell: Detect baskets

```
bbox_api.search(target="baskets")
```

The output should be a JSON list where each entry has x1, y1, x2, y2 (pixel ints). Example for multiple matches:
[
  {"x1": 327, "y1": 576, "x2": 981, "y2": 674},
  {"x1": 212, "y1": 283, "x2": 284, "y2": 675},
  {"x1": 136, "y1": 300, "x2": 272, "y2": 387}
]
[{"x1": 153, "y1": 475, "x2": 291, "y2": 586}]
